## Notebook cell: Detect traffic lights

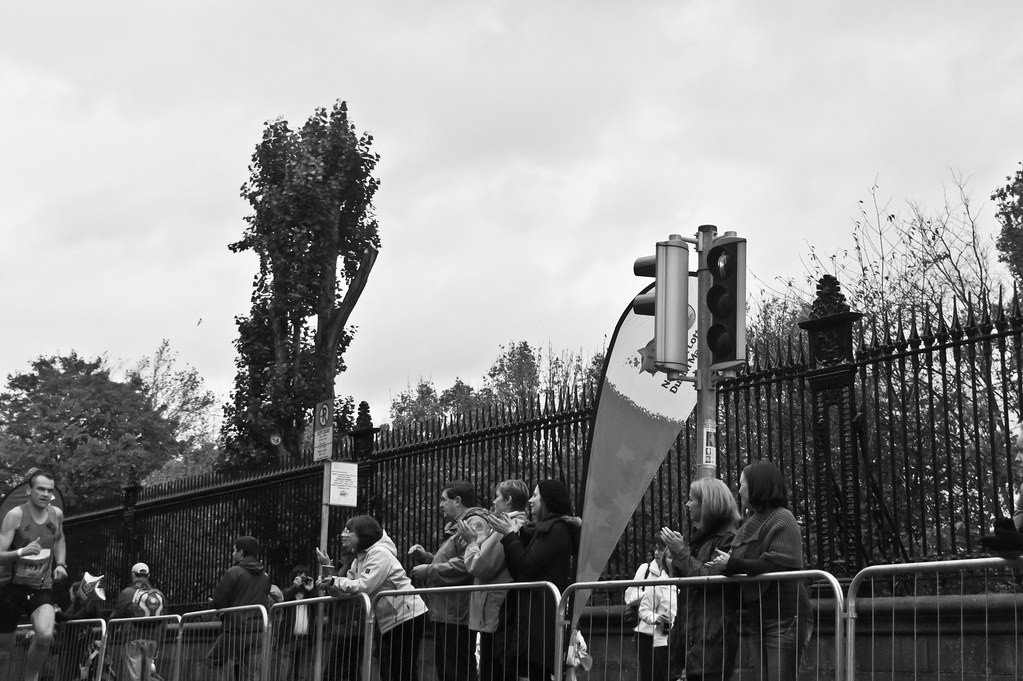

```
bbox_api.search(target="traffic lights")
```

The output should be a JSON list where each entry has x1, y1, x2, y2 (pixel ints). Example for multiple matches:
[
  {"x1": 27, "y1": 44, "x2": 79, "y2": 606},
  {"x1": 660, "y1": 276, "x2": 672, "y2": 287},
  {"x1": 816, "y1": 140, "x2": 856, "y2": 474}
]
[
  {"x1": 632, "y1": 234, "x2": 689, "y2": 375},
  {"x1": 706, "y1": 230, "x2": 748, "y2": 371}
]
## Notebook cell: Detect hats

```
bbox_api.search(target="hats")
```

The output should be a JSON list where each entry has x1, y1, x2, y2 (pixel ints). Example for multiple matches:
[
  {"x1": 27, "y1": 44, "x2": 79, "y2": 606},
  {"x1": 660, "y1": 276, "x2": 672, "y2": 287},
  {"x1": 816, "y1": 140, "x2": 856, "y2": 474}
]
[
  {"x1": 538, "y1": 479, "x2": 570, "y2": 515},
  {"x1": 131, "y1": 562, "x2": 150, "y2": 575}
]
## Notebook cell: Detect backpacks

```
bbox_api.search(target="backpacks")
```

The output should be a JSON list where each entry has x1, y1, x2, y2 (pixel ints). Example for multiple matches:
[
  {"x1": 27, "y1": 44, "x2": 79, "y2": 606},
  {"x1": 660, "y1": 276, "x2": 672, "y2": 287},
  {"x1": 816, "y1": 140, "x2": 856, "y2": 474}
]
[{"x1": 131, "y1": 581, "x2": 165, "y2": 627}]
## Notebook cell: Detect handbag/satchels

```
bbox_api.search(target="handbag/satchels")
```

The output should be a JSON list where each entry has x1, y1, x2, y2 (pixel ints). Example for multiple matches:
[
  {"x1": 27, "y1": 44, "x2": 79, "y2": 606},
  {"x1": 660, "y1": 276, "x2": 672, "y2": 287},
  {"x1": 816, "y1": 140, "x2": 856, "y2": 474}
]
[{"x1": 621, "y1": 600, "x2": 643, "y2": 630}]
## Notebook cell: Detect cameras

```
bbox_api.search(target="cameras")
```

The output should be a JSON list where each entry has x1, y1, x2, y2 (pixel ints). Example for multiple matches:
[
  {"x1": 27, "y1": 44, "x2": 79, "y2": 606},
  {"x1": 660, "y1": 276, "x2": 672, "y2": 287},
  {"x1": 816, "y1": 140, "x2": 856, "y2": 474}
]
[{"x1": 301, "y1": 577, "x2": 309, "y2": 583}]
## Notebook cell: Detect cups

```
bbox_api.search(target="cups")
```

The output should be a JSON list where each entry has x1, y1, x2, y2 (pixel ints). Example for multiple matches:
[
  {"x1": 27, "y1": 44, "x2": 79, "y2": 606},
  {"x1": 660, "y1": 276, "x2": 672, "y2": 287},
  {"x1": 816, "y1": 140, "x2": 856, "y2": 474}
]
[{"x1": 322, "y1": 565, "x2": 335, "y2": 581}]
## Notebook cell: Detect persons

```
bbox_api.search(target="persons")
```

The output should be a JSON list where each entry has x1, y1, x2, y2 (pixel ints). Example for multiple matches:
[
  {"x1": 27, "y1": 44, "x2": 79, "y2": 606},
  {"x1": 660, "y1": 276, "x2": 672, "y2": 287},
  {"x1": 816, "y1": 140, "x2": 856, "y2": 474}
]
[
  {"x1": 408, "y1": 480, "x2": 582, "y2": 681},
  {"x1": 704, "y1": 462, "x2": 814, "y2": 681},
  {"x1": 979, "y1": 422, "x2": 1023, "y2": 559},
  {"x1": 624, "y1": 524, "x2": 680, "y2": 681},
  {"x1": 315, "y1": 515, "x2": 429, "y2": 681},
  {"x1": 0, "y1": 470, "x2": 69, "y2": 681},
  {"x1": 203, "y1": 536, "x2": 317, "y2": 681},
  {"x1": 54, "y1": 581, "x2": 101, "y2": 681},
  {"x1": 660, "y1": 476, "x2": 740, "y2": 681},
  {"x1": 106, "y1": 563, "x2": 167, "y2": 681}
]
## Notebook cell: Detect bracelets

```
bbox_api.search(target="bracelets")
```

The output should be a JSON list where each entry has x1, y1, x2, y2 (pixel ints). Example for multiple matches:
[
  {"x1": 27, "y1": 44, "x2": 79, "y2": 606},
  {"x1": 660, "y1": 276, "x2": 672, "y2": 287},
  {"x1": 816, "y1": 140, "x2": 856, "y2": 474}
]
[
  {"x1": 56, "y1": 563, "x2": 67, "y2": 569},
  {"x1": 18, "y1": 548, "x2": 22, "y2": 556}
]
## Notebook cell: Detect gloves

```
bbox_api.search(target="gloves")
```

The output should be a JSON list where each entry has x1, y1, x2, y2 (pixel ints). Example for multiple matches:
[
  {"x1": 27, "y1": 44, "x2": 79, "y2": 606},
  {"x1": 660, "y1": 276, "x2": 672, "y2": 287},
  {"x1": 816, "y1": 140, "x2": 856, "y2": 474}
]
[
  {"x1": 974, "y1": 528, "x2": 1022, "y2": 552},
  {"x1": 992, "y1": 516, "x2": 1023, "y2": 533}
]
